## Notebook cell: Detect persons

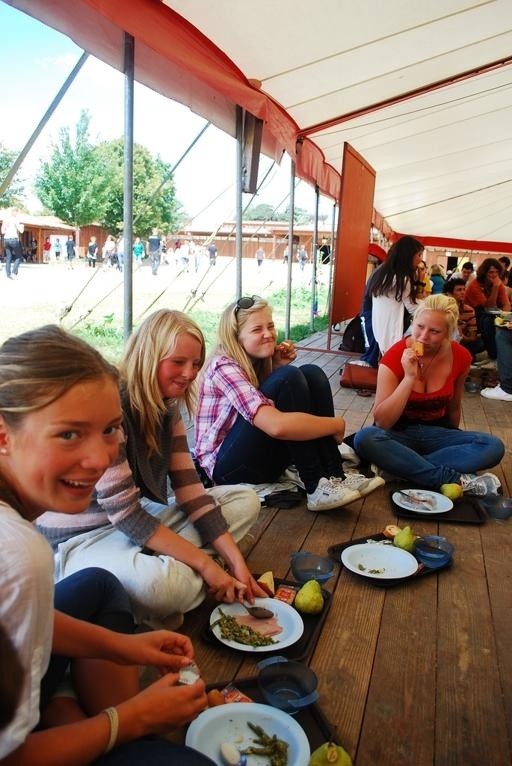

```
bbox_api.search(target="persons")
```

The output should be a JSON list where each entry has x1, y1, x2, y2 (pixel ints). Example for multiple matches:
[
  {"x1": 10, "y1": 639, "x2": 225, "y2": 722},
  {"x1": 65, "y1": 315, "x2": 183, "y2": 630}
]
[
  {"x1": 0, "y1": 324, "x2": 219, "y2": 766},
  {"x1": 34, "y1": 308, "x2": 270, "y2": 631},
  {"x1": 1, "y1": 209, "x2": 24, "y2": 281},
  {"x1": 361, "y1": 236, "x2": 512, "y2": 401},
  {"x1": 193, "y1": 295, "x2": 385, "y2": 511},
  {"x1": 297, "y1": 244, "x2": 309, "y2": 270},
  {"x1": 284, "y1": 246, "x2": 288, "y2": 263},
  {"x1": 23, "y1": 234, "x2": 124, "y2": 271},
  {"x1": 133, "y1": 227, "x2": 218, "y2": 275},
  {"x1": 256, "y1": 247, "x2": 265, "y2": 266},
  {"x1": 353, "y1": 294, "x2": 505, "y2": 498}
]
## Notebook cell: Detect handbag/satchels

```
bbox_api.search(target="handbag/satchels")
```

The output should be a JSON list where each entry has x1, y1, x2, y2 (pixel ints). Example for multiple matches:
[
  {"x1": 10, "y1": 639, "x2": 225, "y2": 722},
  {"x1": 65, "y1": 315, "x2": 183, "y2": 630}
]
[
  {"x1": 340, "y1": 362, "x2": 378, "y2": 390},
  {"x1": 338, "y1": 313, "x2": 366, "y2": 353}
]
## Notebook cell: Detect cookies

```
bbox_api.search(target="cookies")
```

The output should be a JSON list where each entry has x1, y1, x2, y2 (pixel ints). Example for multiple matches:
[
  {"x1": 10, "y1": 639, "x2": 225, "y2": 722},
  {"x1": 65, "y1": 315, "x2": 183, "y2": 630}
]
[{"x1": 411, "y1": 342, "x2": 425, "y2": 356}]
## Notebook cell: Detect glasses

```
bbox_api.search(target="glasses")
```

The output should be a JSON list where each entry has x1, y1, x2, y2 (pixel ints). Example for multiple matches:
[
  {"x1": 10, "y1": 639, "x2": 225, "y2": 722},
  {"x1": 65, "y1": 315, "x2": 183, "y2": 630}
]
[{"x1": 234, "y1": 295, "x2": 262, "y2": 316}]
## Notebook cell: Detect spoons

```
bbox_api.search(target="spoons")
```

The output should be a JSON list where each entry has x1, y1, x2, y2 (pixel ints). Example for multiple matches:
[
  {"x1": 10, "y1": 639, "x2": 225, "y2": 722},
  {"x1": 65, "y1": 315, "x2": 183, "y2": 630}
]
[
  {"x1": 394, "y1": 488, "x2": 432, "y2": 511},
  {"x1": 235, "y1": 598, "x2": 274, "y2": 619}
]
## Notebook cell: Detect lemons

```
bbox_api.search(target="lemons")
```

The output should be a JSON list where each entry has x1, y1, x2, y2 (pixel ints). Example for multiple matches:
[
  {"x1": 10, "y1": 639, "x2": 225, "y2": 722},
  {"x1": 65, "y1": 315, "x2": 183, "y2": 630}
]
[{"x1": 493, "y1": 318, "x2": 504, "y2": 324}]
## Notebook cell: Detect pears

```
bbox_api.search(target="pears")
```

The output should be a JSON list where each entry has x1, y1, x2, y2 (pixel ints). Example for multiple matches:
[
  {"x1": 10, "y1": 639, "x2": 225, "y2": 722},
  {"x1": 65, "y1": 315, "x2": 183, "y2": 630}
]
[
  {"x1": 294, "y1": 580, "x2": 323, "y2": 611},
  {"x1": 441, "y1": 483, "x2": 465, "y2": 498},
  {"x1": 310, "y1": 742, "x2": 352, "y2": 766},
  {"x1": 392, "y1": 525, "x2": 419, "y2": 552}
]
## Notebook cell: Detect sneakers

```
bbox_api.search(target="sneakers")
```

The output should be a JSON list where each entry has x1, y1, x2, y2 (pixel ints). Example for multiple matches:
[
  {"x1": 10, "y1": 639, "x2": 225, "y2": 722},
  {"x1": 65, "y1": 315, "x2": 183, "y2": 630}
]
[
  {"x1": 339, "y1": 472, "x2": 385, "y2": 497},
  {"x1": 366, "y1": 462, "x2": 408, "y2": 484},
  {"x1": 466, "y1": 358, "x2": 512, "y2": 402},
  {"x1": 459, "y1": 473, "x2": 504, "y2": 502},
  {"x1": 306, "y1": 476, "x2": 362, "y2": 512}
]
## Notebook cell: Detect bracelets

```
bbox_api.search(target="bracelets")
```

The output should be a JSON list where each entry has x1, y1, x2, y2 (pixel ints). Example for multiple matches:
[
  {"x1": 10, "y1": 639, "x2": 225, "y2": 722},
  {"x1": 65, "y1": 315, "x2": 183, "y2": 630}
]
[{"x1": 102, "y1": 706, "x2": 119, "y2": 754}]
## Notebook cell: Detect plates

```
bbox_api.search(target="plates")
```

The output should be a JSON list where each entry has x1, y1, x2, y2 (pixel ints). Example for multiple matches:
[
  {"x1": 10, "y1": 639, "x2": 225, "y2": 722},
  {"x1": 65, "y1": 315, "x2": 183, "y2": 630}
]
[
  {"x1": 341, "y1": 544, "x2": 418, "y2": 579},
  {"x1": 210, "y1": 597, "x2": 304, "y2": 652},
  {"x1": 392, "y1": 489, "x2": 453, "y2": 513},
  {"x1": 186, "y1": 703, "x2": 310, "y2": 765}
]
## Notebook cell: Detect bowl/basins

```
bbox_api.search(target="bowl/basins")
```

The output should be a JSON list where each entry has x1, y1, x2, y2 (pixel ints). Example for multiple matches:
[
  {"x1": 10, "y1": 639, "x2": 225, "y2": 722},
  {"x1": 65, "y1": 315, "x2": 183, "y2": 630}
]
[
  {"x1": 290, "y1": 552, "x2": 334, "y2": 583},
  {"x1": 257, "y1": 656, "x2": 319, "y2": 714},
  {"x1": 415, "y1": 535, "x2": 455, "y2": 569},
  {"x1": 478, "y1": 496, "x2": 511, "y2": 518}
]
